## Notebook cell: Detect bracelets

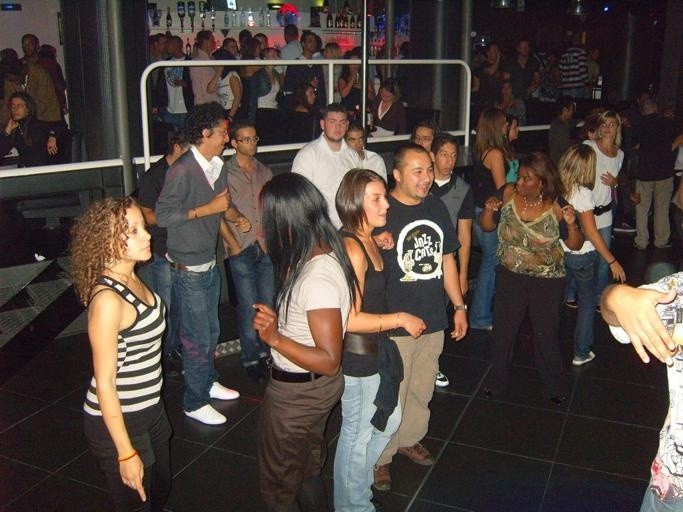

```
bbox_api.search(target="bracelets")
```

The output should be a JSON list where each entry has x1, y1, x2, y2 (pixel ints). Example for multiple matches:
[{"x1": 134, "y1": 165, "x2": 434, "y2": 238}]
[
  {"x1": 611, "y1": 182, "x2": 621, "y2": 190},
  {"x1": 227, "y1": 113, "x2": 235, "y2": 120},
  {"x1": 607, "y1": 258, "x2": 618, "y2": 265},
  {"x1": 376, "y1": 312, "x2": 384, "y2": 336},
  {"x1": 565, "y1": 223, "x2": 581, "y2": 232},
  {"x1": 190, "y1": 207, "x2": 202, "y2": 224},
  {"x1": 395, "y1": 312, "x2": 400, "y2": 329},
  {"x1": 117, "y1": 448, "x2": 142, "y2": 465}
]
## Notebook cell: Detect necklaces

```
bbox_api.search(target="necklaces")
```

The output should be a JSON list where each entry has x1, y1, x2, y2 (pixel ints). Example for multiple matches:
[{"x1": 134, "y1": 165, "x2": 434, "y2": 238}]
[{"x1": 514, "y1": 195, "x2": 547, "y2": 217}]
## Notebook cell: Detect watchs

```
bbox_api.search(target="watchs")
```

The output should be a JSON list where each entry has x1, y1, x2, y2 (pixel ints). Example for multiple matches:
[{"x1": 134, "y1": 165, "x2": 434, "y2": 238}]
[
  {"x1": 47, "y1": 133, "x2": 60, "y2": 139},
  {"x1": 451, "y1": 303, "x2": 471, "y2": 313}
]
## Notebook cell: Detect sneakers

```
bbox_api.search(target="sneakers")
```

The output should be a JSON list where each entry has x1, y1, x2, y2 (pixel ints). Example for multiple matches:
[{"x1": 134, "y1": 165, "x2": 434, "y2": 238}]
[
  {"x1": 179, "y1": 402, "x2": 229, "y2": 428},
  {"x1": 570, "y1": 350, "x2": 596, "y2": 367},
  {"x1": 209, "y1": 378, "x2": 239, "y2": 404},
  {"x1": 433, "y1": 370, "x2": 450, "y2": 392}
]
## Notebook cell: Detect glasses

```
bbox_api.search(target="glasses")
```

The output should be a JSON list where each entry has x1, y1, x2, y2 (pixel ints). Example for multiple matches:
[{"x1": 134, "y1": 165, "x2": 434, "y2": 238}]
[
  {"x1": 412, "y1": 134, "x2": 436, "y2": 146},
  {"x1": 233, "y1": 135, "x2": 262, "y2": 145},
  {"x1": 345, "y1": 135, "x2": 365, "y2": 143},
  {"x1": 596, "y1": 121, "x2": 619, "y2": 131}
]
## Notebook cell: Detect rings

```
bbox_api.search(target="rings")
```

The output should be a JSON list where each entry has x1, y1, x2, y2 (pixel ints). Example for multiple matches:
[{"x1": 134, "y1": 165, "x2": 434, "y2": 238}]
[{"x1": 48, "y1": 146, "x2": 54, "y2": 150}]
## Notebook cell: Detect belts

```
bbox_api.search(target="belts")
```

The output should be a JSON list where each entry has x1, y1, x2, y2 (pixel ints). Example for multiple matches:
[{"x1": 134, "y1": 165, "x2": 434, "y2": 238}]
[{"x1": 263, "y1": 365, "x2": 326, "y2": 385}]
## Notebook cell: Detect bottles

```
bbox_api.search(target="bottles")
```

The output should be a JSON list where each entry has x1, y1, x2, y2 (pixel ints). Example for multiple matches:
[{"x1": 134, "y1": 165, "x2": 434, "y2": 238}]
[
  {"x1": 231, "y1": 7, "x2": 237, "y2": 26},
  {"x1": 266, "y1": 8, "x2": 271, "y2": 26},
  {"x1": 343, "y1": 11, "x2": 348, "y2": 27},
  {"x1": 188, "y1": 1, "x2": 195, "y2": 27},
  {"x1": 166, "y1": 7, "x2": 172, "y2": 28},
  {"x1": 199, "y1": 2, "x2": 206, "y2": 26},
  {"x1": 336, "y1": 10, "x2": 340, "y2": 27},
  {"x1": 210, "y1": 5, "x2": 216, "y2": 26},
  {"x1": 241, "y1": 8, "x2": 245, "y2": 27},
  {"x1": 350, "y1": 11, "x2": 355, "y2": 28},
  {"x1": 340, "y1": 9, "x2": 344, "y2": 27},
  {"x1": 357, "y1": 9, "x2": 362, "y2": 28},
  {"x1": 178, "y1": 2, "x2": 185, "y2": 27},
  {"x1": 365, "y1": 103, "x2": 372, "y2": 132},
  {"x1": 354, "y1": 104, "x2": 362, "y2": 126},
  {"x1": 224, "y1": 8, "x2": 229, "y2": 26},
  {"x1": 259, "y1": 7, "x2": 264, "y2": 27},
  {"x1": 327, "y1": 8, "x2": 333, "y2": 27}
]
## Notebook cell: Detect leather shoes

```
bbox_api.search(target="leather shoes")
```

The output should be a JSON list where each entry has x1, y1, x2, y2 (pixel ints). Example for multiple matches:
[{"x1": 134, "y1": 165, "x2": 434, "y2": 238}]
[
  {"x1": 369, "y1": 461, "x2": 395, "y2": 494},
  {"x1": 257, "y1": 357, "x2": 273, "y2": 375},
  {"x1": 397, "y1": 440, "x2": 438, "y2": 467},
  {"x1": 246, "y1": 360, "x2": 267, "y2": 384}
]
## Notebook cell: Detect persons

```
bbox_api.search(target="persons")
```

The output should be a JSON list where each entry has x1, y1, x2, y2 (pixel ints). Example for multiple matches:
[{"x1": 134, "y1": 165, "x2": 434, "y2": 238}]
[
  {"x1": 310, "y1": 75, "x2": 325, "y2": 100},
  {"x1": 471, "y1": 38, "x2": 511, "y2": 83},
  {"x1": 189, "y1": 31, "x2": 219, "y2": 105},
  {"x1": 154, "y1": 33, "x2": 168, "y2": 67},
  {"x1": 494, "y1": 80, "x2": 527, "y2": 126},
  {"x1": 281, "y1": 31, "x2": 327, "y2": 103},
  {"x1": 476, "y1": 149, "x2": 585, "y2": 407},
  {"x1": 245, "y1": 47, "x2": 285, "y2": 144},
  {"x1": 619, "y1": 86, "x2": 654, "y2": 227},
  {"x1": 611, "y1": 186, "x2": 635, "y2": 232},
  {"x1": 1, "y1": 89, "x2": 72, "y2": 168},
  {"x1": 65, "y1": 193, "x2": 169, "y2": 512},
  {"x1": 250, "y1": 170, "x2": 366, "y2": 512},
  {"x1": 598, "y1": 272, "x2": 683, "y2": 512},
  {"x1": 467, "y1": 103, "x2": 513, "y2": 331},
  {"x1": 151, "y1": 101, "x2": 252, "y2": 425},
  {"x1": 236, "y1": 28, "x2": 252, "y2": 47},
  {"x1": 547, "y1": 94, "x2": 573, "y2": 167},
  {"x1": 0, "y1": 47, "x2": 20, "y2": 122},
  {"x1": 587, "y1": 45, "x2": 602, "y2": 91},
  {"x1": 278, "y1": 22, "x2": 305, "y2": 72},
  {"x1": 150, "y1": 37, "x2": 160, "y2": 89},
  {"x1": 239, "y1": 38, "x2": 263, "y2": 76},
  {"x1": 428, "y1": 132, "x2": 475, "y2": 387},
  {"x1": 36, "y1": 42, "x2": 70, "y2": 117},
  {"x1": 253, "y1": 32, "x2": 269, "y2": 73},
  {"x1": 205, "y1": 49, "x2": 244, "y2": 124},
  {"x1": 618, "y1": 97, "x2": 681, "y2": 251},
  {"x1": 408, "y1": 118, "x2": 438, "y2": 159},
  {"x1": 286, "y1": 102, "x2": 365, "y2": 233},
  {"x1": 337, "y1": 49, "x2": 375, "y2": 112},
  {"x1": 661, "y1": 105, "x2": 683, "y2": 248},
  {"x1": 343, "y1": 119, "x2": 388, "y2": 193},
  {"x1": 568, "y1": 115, "x2": 641, "y2": 206},
  {"x1": 559, "y1": 144, "x2": 626, "y2": 367},
  {"x1": 136, "y1": 121, "x2": 194, "y2": 383},
  {"x1": 372, "y1": 141, "x2": 468, "y2": 493},
  {"x1": 332, "y1": 168, "x2": 427, "y2": 512},
  {"x1": 508, "y1": 38, "x2": 540, "y2": 99},
  {"x1": 280, "y1": 83, "x2": 324, "y2": 142},
  {"x1": 220, "y1": 37, "x2": 239, "y2": 60},
  {"x1": 323, "y1": 41, "x2": 344, "y2": 105},
  {"x1": 19, "y1": 33, "x2": 73, "y2": 144},
  {"x1": 367, "y1": 77, "x2": 409, "y2": 135},
  {"x1": 563, "y1": 107, "x2": 624, "y2": 312},
  {"x1": 151, "y1": 36, "x2": 195, "y2": 130},
  {"x1": 559, "y1": 33, "x2": 592, "y2": 100},
  {"x1": 219, "y1": 120, "x2": 278, "y2": 383},
  {"x1": 392, "y1": 40, "x2": 418, "y2": 83},
  {"x1": 507, "y1": 112, "x2": 524, "y2": 187}
]
